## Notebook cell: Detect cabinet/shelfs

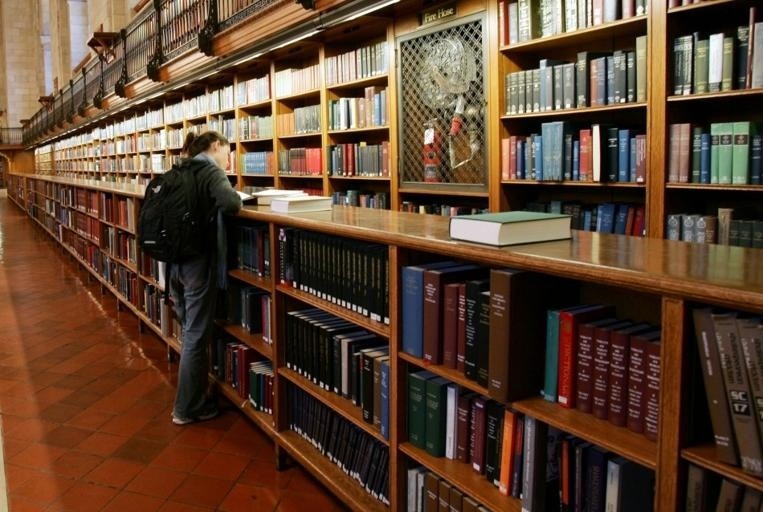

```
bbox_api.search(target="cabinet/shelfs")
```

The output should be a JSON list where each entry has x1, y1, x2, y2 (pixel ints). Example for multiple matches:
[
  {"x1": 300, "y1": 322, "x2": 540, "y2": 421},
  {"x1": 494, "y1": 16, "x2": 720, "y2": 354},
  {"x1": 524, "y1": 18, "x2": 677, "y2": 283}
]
[{"x1": 9, "y1": 0, "x2": 763, "y2": 512}]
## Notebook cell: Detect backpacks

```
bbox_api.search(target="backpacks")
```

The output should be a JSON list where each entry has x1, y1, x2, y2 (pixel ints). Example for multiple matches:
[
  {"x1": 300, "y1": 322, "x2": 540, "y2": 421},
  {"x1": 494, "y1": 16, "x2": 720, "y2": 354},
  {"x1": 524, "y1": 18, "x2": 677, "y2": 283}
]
[{"x1": 139, "y1": 158, "x2": 215, "y2": 265}]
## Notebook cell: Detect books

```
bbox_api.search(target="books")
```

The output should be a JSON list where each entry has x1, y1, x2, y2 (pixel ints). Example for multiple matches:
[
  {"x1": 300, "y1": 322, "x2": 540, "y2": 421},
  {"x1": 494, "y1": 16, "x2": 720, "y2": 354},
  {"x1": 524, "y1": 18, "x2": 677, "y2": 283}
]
[
  {"x1": 285, "y1": 305, "x2": 391, "y2": 438},
  {"x1": 400, "y1": 201, "x2": 487, "y2": 216},
  {"x1": 278, "y1": 229, "x2": 390, "y2": 325},
  {"x1": 405, "y1": 369, "x2": 654, "y2": 511},
  {"x1": 402, "y1": 259, "x2": 662, "y2": 440},
  {"x1": 505, "y1": 35, "x2": 648, "y2": 114},
  {"x1": 150, "y1": 96, "x2": 194, "y2": 171},
  {"x1": 668, "y1": 1, "x2": 763, "y2": 94},
  {"x1": 30, "y1": 180, "x2": 181, "y2": 340},
  {"x1": 501, "y1": 122, "x2": 646, "y2": 183},
  {"x1": 517, "y1": 199, "x2": 645, "y2": 237},
  {"x1": 208, "y1": 225, "x2": 274, "y2": 415},
  {"x1": 667, "y1": 208, "x2": 763, "y2": 249},
  {"x1": 288, "y1": 383, "x2": 390, "y2": 504},
  {"x1": 237, "y1": 74, "x2": 274, "y2": 193},
  {"x1": 251, "y1": 189, "x2": 332, "y2": 213},
  {"x1": 106, "y1": 109, "x2": 149, "y2": 184},
  {"x1": 667, "y1": 122, "x2": 763, "y2": 184},
  {"x1": 276, "y1": 63, "x2": 323, "y2": 197},
  {"x1": 448, "y1": 212, "x2": 574, "y2": 247},
  {"x1": 499, "y1": 0, "x2": 648, "y2": 46},
  {"x1": 324, "y1": 40, "x2": 390, "y2": 210},
  {"x1": 686, "y1": 307, "x2": 763, "y2": 511},
  {"x1": 34, "y1": 126, "x2": 106, "y2": 181},
  {"x1": 195, "y1": 87, "x2": 237, "y2": 188}
]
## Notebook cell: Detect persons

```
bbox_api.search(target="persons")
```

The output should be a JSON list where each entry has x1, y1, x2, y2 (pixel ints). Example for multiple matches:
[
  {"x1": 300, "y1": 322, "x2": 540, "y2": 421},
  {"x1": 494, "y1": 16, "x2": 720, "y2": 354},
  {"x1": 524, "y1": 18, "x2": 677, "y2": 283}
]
[{"x1": 167, "y1": 131, "x2": 244, "y2": 427}]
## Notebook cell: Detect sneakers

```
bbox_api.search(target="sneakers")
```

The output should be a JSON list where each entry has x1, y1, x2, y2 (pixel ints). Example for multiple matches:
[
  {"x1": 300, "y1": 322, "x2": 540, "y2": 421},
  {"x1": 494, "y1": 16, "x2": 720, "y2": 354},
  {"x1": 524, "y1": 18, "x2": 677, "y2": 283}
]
[{"x1": 172, "y1": 400, "x2": 218, "y2": 426}]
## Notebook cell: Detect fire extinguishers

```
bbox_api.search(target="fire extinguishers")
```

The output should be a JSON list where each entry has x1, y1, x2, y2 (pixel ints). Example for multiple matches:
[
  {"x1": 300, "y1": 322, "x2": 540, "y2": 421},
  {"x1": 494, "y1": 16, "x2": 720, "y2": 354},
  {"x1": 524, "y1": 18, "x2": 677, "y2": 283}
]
[{"x1": 423, "y1": 118, "x2": 443, "y2": 183}]
